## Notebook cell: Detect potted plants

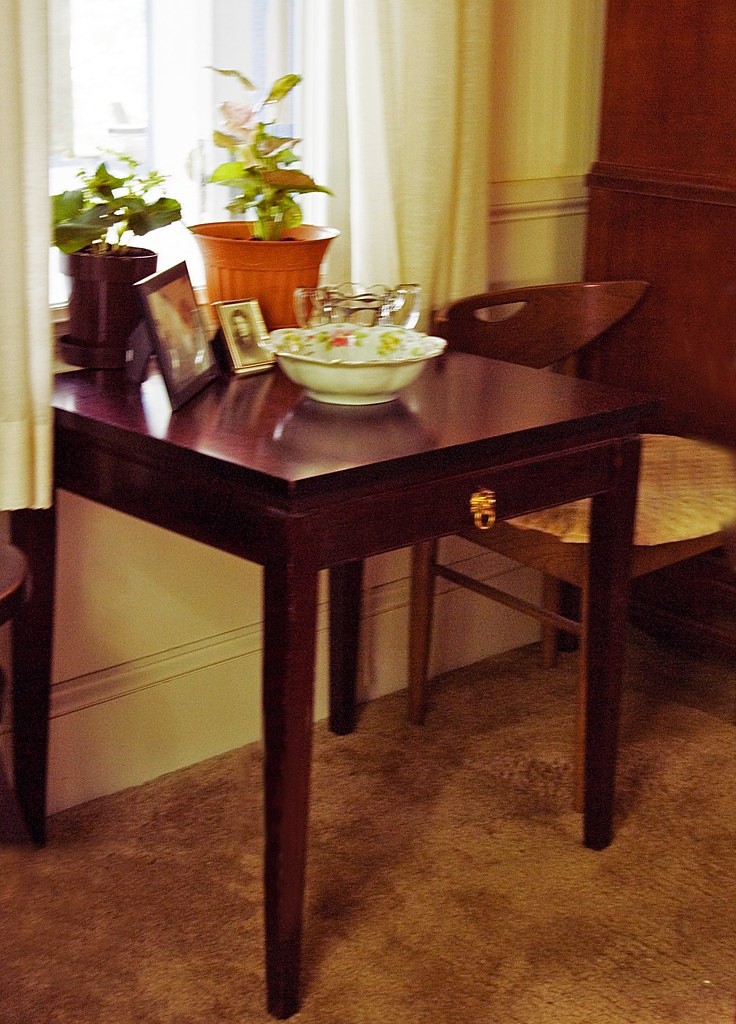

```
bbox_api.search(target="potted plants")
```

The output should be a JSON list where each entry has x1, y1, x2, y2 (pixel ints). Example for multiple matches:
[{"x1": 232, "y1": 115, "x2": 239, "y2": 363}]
[{"x1": 49, "y1": 146, "x2": 182, "y2": 368}]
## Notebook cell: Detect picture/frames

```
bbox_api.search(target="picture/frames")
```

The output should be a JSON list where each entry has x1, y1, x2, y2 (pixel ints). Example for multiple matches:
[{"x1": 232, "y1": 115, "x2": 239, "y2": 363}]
[
  {"x1": 132, "y1": 259, "x2": 222, "y2": 412},
  {"x1": 211, "y1": 299, "x2": 277, "y2": 377}
]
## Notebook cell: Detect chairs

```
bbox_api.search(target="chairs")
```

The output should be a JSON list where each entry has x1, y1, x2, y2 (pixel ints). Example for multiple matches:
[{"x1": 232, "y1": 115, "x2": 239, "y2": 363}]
[{"x1": 407, "y1": 279, "x2": 736, "y2": 816}]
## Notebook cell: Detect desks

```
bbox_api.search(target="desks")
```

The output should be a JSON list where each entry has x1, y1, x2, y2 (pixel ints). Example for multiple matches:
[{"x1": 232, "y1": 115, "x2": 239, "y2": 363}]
[{"x1": 6, "y1": 350, "x2": 657, "y2": 1019}]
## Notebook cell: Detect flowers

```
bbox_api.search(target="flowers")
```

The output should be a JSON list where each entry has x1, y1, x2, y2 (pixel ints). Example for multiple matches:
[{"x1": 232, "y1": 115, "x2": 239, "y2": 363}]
[{"x1": 201, "y1": 62, "x2": 335, "y2": 244}]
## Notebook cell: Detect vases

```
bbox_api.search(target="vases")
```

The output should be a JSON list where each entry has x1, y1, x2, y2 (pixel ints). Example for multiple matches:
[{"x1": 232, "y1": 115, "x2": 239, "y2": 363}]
[{"x1": 187, "y1": 220, "x2": 341, "y2": 333}]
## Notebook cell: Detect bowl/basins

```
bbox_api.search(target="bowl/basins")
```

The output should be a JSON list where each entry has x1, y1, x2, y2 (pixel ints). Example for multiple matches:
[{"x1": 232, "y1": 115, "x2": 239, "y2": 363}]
[
  {"x1": 256, "y1": 323, "x2": 447, "y2": 406},
  {"x1": 293, "y1": 282, "x2": 423, "y2": 328}
]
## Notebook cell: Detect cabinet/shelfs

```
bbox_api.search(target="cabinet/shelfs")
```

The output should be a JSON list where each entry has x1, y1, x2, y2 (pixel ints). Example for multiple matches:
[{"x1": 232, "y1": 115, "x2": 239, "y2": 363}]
[{"x1": 580, "y1": 0, "x2": 736, "y2": 690}]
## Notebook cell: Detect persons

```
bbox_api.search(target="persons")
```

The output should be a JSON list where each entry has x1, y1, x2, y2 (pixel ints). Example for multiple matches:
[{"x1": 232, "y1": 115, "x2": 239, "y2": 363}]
[{"x1": 230, "y1": 309, "x2": 263, "y2": 363}]
